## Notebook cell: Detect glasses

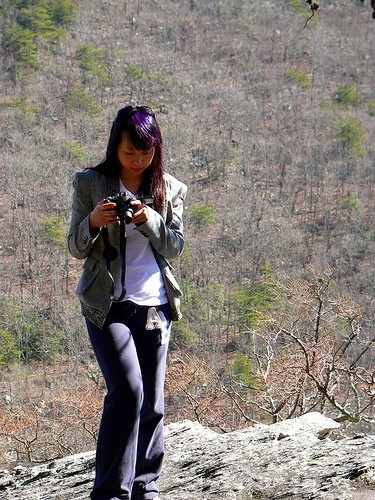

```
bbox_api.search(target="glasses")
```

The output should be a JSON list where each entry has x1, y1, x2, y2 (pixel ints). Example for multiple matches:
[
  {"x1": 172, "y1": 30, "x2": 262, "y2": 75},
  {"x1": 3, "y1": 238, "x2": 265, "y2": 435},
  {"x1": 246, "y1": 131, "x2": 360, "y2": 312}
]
[{"x1": 118, "y1": 105, "x2": 153, "y2": 117}]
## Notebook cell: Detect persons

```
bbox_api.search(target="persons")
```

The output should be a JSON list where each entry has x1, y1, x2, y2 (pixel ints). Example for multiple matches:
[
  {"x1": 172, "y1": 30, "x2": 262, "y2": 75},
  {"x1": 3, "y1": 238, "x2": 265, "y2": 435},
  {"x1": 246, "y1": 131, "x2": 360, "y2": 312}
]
[{"x1": 65, "y1": 105, "x2": 190, "y2": 500}]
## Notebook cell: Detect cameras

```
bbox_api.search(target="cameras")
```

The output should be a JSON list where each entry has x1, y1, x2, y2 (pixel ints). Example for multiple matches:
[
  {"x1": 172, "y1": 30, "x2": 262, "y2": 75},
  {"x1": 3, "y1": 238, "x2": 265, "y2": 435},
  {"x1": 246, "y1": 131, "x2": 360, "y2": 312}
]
[{"x1": 102, "y1": 191, "x2": 134, "y2": 225}]
